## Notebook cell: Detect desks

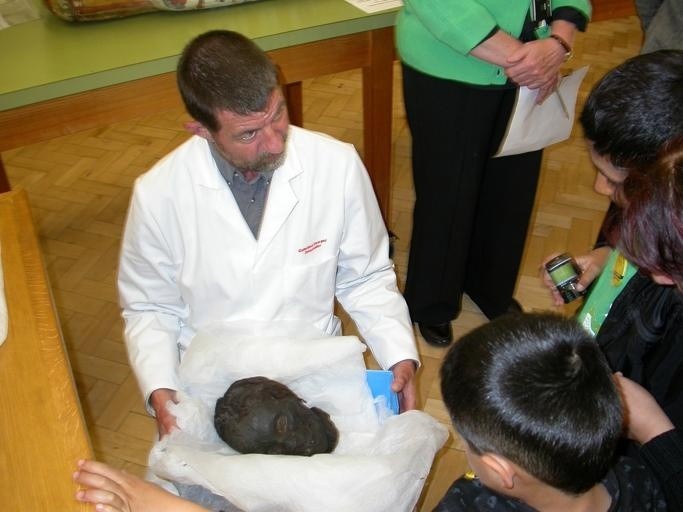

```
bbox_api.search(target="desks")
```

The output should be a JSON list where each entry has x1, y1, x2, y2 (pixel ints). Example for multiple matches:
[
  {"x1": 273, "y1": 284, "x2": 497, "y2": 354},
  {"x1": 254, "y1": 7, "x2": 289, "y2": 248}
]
[
  {"x1": 0, "y1": 1, "x2": 404, "y2": 230},
  {"x1": 0, "y1": 189, "x2": 98, "y2": 512}
]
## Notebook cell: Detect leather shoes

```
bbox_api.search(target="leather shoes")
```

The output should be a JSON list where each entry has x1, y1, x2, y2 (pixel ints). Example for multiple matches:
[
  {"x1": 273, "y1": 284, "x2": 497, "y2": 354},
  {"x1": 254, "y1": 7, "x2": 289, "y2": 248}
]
[
  {"x1": 468, "y1": 290, "x2": 522, "y2": 320},
  {"x1": 417, "y1": 321, "x2": 453, "y2": 346}
]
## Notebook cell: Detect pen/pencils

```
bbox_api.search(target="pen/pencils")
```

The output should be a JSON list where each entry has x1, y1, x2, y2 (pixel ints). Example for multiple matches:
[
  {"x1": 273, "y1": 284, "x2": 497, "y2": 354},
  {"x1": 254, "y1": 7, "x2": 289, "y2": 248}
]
[{"x1": 555, "y1": 89, "x2": 569, "y2": 120}]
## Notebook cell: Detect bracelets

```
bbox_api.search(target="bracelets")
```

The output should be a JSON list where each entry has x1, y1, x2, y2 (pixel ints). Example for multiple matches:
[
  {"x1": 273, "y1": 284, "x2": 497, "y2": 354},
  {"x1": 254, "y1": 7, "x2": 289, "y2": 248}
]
[{"x1": 550, "y1": 33, "x2": 576, "y2": 65}]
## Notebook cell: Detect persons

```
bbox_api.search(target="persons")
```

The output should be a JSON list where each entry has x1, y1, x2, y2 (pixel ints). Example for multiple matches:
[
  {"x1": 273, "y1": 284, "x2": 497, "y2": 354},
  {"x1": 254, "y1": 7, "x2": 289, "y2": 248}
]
[
  {"x1": 214, "y1": 376, "x2": 338, "y2": 457},
  {"x1": 540, "y1": 48, "x2": 682, "y2": 337},
  {"x1": 116, "y1": 28, "x2": 423, "y2": 444},
  {"x1": 632, "y1": 2, "x2": 682, "y2": 57},
  {"x1": 394, "y1": 1, "x2": 593, "y2": 348},
  {"x1": 597, "y1": 134, "x2": 682, "y2": 512},
  {"x1": 71, "y1": 309, "x2": 670, "y2": 511}
]
